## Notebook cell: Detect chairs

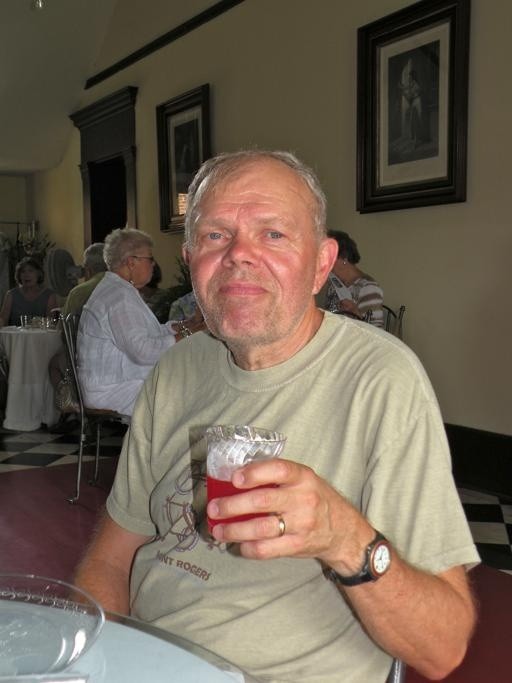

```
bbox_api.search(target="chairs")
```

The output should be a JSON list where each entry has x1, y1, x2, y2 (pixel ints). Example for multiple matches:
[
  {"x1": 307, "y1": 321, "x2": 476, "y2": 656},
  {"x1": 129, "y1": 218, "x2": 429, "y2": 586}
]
[
  {"x1": 379, "y1": 302, "x2": 406, "y2": 342},
  {"x1": 332, "y1": 306, "x2": 373, "y2": 325},
  {"x1": 58, "y1": 310, "x2": 132, "y2": 503}
]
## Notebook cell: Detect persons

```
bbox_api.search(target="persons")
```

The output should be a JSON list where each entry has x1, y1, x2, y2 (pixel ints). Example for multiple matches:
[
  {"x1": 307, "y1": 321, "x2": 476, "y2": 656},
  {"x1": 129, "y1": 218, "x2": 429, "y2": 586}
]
[
  {"x1": 48, "y1": 242, "x2": 115, "y2": 427},
  {"x1": 80, "y1": 226, "x2": 206, "y2": 423},
  {"x1": 0, "y1": 256, "x2": 197, "y2": 323},
  {"x1": 60, "y1": 147, "x2": 480, "y2": 683},
  {"x1": 322, "y1": 225, "x2": 386, "y2": 328}
]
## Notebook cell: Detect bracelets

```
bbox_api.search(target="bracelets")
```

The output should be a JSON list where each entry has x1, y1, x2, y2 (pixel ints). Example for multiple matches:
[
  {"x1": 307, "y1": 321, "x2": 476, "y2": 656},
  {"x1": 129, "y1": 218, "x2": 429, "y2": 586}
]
[
  {"x1": 178, "y1": 319, "x2": 185, "y2": 329},
  {"x1": 180, "y1": 327, "x2": 193, "y2": 338}
]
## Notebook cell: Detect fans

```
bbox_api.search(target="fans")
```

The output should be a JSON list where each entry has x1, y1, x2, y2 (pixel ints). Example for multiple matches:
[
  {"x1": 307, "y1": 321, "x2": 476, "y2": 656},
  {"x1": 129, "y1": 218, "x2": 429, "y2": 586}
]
[{"x1": 44, "y1": 246, "x2": 85, "y2": 300}]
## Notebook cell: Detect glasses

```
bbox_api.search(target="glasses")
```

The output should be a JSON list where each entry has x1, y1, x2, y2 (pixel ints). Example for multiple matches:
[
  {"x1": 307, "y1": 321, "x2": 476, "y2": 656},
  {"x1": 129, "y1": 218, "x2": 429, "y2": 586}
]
[{"x1": 132, "y1": 255, "x2": 154, "y2": 263}]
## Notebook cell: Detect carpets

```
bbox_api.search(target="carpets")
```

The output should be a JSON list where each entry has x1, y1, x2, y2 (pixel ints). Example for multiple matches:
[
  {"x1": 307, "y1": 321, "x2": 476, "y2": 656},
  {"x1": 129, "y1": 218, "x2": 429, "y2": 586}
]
[
  {"x1": 450, "y1": 463, "x2": 512, "y2": 578},
  {"x1": 0, "y1": 414, "x2": 126, "y2": 477}
]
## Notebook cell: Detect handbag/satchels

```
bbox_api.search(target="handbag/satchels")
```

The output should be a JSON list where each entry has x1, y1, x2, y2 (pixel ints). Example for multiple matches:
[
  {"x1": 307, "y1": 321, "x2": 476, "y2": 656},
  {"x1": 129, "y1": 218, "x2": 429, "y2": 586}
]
[{"x1": 49, "y1": 347, "x2": 80, "y2": 414}]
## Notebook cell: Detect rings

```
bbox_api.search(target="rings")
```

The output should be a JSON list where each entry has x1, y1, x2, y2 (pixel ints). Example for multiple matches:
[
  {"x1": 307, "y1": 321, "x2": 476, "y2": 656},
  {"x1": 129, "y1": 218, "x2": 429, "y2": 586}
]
[{"x1": 275, "y1": 513, "x2": 287, "y2": 537}]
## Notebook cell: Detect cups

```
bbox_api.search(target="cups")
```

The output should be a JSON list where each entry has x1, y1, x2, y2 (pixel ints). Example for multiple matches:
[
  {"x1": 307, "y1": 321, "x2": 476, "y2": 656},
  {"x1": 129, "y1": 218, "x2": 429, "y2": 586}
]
[
  {"x1": 202, "y1": 422, "x2": 287, "y2": 536},
  {"x1": 19, "y1": 313, "x2": 54, "y2": 330}
]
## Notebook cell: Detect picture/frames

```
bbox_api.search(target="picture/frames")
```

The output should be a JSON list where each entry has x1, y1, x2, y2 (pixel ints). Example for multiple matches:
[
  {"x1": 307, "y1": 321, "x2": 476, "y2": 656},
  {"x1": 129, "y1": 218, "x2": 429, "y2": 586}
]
[
  {"x1": 354, "y1": 1, "x2": 472, "y2": 213},
  {"x1": 154, "y1": 78, "x2": 213, "y2": 240}
]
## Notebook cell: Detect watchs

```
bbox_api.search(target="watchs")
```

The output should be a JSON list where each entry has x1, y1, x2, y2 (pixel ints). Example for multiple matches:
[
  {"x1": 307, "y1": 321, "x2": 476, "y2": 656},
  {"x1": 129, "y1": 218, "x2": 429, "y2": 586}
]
[{"x1": 324, "y1": 527, "x2": 394, "y2": 585}]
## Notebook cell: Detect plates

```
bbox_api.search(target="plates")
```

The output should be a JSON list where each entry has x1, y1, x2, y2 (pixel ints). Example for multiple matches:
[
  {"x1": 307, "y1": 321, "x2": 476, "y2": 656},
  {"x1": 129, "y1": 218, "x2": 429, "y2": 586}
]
[{"x1": 0, "y1": 571, "x2": 108, "y2": 681}]
